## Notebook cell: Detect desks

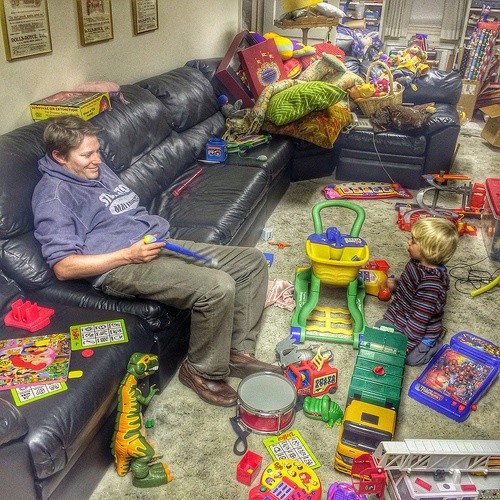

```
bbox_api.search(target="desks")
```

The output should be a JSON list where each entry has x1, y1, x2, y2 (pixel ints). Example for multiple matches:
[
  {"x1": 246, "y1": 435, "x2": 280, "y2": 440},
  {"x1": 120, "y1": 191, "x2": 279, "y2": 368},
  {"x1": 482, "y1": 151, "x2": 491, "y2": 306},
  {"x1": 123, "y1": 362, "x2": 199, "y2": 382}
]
[{"x1": 274, "y1": 18, "x2": 339, "y2": 46}]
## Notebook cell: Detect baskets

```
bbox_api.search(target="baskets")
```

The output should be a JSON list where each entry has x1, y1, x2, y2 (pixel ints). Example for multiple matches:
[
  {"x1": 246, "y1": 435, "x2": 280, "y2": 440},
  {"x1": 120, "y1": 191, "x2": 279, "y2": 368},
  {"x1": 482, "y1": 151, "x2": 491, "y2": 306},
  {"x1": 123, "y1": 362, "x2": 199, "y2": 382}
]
[{"x1": 354, "y1": 61, "x2": 405, "y2": 118}]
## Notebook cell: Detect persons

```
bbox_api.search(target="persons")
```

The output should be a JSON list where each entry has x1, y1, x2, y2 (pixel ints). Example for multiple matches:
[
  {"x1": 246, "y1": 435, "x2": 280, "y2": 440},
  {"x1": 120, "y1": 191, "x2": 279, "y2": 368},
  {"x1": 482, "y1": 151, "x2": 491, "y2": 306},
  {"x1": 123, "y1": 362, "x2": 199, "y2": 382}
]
[
  {"x1": 374, "y1": 217, "x2": 459, "y2": 366},
  {"x1": 32, "y1": 115, "x2": 285, "y2": 407}
]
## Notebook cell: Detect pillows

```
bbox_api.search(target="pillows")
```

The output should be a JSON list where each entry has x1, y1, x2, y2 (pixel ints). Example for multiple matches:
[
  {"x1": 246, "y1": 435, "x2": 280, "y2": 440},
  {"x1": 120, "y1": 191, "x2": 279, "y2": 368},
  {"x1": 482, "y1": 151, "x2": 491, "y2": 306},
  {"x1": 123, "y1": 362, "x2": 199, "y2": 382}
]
[
  {"x1": 261, "y1": 101, "x2": 355, "y2": 148},
  {"x1": 311, "y1": 3, "x2": 346, "y2": 18},
  {"x1": 267, "y1": 82, "x2": 347, "y2": 126}
]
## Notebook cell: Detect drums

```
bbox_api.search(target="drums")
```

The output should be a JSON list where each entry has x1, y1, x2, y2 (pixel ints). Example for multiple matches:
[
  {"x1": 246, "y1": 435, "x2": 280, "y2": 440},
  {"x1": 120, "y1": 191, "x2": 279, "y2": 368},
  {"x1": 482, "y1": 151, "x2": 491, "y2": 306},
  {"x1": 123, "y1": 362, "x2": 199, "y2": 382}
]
[{"x1": 235, "y1": 370, "x2": 298, "y2": 437}]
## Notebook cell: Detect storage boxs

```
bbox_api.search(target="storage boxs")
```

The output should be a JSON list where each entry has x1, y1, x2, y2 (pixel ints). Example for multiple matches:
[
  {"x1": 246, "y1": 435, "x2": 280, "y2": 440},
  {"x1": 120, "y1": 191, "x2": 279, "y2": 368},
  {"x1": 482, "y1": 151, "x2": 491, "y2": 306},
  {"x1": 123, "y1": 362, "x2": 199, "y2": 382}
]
[
  {"x1": 215, "y1": 30, "x2": 287, "y2": 109},
  {"x1": 457, "y1": 79, "x2": 480, "y2": 119},
  {"x1": 479, "y1": 105, "x2": 500, "y2": 147},
  {"x1": 29, "y1": 91, "x2": 111, "y2": 121},
  {"x1": 480, "y1": 178, "x2": 500, "y2": 262}
]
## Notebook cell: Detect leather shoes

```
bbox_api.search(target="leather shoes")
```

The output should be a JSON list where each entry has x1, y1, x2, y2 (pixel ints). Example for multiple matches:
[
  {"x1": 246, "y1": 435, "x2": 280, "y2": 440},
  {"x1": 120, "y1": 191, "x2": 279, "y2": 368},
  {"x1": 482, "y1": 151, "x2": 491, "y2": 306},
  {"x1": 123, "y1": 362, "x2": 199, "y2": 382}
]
[
  {"x1": 228, "y1": 351, "x2": 283, "y2": 379},
  {"x1": 178, "y1": 360, "x2": 238, "y2": 407}
]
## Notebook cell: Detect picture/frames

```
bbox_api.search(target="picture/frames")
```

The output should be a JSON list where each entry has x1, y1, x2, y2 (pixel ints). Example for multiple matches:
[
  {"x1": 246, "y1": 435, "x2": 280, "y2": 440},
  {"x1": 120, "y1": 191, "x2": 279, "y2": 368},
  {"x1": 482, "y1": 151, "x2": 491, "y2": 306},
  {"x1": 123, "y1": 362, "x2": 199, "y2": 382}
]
[
  {"x1": 130, "y1": 0, "x2": 159, "y2": 36},
  {"x1": 77, "y1": 0, "x2": 114, "y2": 46},
  {"x1": 0, "y1": 0, "x2": 53, "y2": 61}
]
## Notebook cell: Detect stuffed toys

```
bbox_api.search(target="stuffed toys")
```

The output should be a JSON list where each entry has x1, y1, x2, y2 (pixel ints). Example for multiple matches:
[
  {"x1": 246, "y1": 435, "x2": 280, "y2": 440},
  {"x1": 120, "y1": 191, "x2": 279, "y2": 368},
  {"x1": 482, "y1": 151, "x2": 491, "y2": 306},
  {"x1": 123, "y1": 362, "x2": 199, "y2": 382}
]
[{"x1": 392, "y1": 38, "x2": 429, "y2": 76}]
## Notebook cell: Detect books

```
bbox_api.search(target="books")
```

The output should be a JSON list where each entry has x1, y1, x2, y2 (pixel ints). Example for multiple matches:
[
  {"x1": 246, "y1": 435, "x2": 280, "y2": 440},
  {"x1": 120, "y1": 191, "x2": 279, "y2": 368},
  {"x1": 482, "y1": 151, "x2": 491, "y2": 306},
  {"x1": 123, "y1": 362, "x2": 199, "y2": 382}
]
[{"x1": 413, "y1": 34, "x2": 428, "y2": 51}]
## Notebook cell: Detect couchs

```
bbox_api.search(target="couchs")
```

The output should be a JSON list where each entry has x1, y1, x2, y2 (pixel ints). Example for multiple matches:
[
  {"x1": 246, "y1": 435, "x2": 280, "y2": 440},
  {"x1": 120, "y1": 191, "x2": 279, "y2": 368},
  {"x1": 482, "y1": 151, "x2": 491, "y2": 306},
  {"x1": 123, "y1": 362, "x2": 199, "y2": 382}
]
[{"x1": 0, "y1": 57, "x2": 462, "y2": 500}]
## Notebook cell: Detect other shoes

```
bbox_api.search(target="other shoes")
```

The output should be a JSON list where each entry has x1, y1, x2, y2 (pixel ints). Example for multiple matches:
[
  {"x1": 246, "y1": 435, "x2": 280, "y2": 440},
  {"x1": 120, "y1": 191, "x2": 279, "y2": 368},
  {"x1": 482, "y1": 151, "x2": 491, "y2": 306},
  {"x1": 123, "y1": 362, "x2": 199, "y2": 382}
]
[{"x1": 437, "y1": 326, "x2": 448, "y2": 342}]
